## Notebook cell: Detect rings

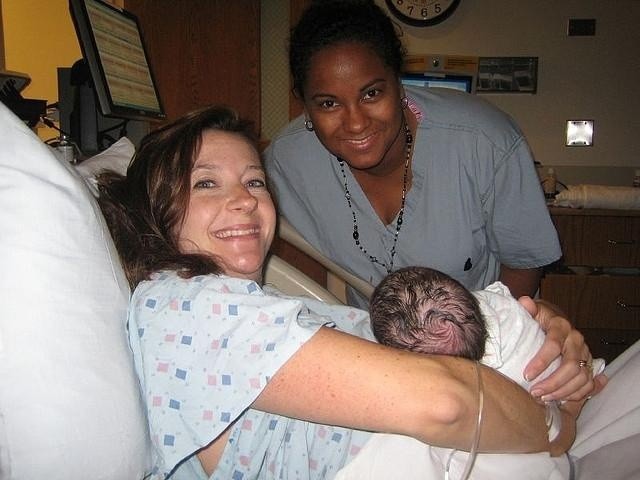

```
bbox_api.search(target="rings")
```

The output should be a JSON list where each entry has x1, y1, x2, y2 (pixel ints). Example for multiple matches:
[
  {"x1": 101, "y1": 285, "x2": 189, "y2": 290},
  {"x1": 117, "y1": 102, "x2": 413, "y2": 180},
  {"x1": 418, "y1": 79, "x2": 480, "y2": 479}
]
[{"x1": 576, "y1": 358, "x2": 593, "y2": 369}]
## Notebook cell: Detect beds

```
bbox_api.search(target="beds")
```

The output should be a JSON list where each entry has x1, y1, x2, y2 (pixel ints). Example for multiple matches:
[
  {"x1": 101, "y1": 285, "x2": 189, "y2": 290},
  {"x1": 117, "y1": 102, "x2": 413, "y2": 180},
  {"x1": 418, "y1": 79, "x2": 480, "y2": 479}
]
[{"x1": 0, "y1": 107, "x2": 384, "y2": 480}]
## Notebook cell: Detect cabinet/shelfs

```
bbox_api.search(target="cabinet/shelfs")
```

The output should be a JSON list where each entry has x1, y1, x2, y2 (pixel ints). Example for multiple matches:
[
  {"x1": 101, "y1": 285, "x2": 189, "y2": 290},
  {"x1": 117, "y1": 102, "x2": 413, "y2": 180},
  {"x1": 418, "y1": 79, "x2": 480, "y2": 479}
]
[{"x1": 540, "y1": 208, "x2": 639, "y2": 368}]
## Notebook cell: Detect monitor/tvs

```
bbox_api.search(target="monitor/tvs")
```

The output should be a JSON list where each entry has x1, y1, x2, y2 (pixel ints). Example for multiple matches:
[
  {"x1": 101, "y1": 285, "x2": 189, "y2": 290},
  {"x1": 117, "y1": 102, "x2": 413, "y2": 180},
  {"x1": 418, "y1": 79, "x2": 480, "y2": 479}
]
[
  {"x1": 68, "y1": 0, "x2": 168, "y2": 123},
  {"x1": 403, "y1": 76, "x2": 472, "y2": 93}
]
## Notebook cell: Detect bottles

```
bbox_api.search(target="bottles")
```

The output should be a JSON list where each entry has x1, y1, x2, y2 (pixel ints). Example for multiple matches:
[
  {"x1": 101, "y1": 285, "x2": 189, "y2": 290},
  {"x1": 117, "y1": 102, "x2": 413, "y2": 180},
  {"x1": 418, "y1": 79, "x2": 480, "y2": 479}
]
[
  {"x1": 544, "y1": 168, "x2": 556, "y2": 199},
  {"x1": 632, "y1": 169, "x2": 640, "y2": 189},
  {"x1": 56, "y1": 135, "x2": 75, "y2": 166}
]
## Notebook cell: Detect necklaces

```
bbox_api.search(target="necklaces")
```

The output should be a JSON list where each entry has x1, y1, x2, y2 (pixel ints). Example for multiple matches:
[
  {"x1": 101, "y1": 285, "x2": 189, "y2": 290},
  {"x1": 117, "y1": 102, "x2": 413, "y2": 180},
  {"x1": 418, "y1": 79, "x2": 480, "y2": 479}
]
[{"x1": 338, "y1": 110, "x2": 413, "y2": 275}]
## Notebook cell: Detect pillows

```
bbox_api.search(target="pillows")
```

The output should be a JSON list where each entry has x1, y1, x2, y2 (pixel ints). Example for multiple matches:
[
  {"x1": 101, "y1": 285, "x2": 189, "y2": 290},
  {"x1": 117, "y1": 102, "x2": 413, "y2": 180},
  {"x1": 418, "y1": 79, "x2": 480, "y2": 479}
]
[{"x1": 0, "y1": 101, "x2": 151, "y2": 480}]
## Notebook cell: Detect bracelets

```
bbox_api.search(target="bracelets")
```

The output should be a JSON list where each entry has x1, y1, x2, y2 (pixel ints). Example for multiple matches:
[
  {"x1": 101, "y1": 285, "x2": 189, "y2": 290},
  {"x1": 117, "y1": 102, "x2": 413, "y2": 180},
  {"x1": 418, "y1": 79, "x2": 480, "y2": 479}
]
[{"x1": 546, "y1": 400, "x2": 566, "y2": 443}]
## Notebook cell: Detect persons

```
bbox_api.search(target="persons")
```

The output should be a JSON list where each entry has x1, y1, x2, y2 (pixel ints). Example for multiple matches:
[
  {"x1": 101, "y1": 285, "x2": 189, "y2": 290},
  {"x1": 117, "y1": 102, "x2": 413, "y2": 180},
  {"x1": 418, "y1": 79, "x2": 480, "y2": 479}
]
[
  {"x1": 95, "y1": 102, "x2": 609, "y2": 480},
  {"x1": 369, "y1": 267, "x2": 606, "y2": 406},
  {"x1": 258, "y1": 0, "x2": 562, "y2": 303}
]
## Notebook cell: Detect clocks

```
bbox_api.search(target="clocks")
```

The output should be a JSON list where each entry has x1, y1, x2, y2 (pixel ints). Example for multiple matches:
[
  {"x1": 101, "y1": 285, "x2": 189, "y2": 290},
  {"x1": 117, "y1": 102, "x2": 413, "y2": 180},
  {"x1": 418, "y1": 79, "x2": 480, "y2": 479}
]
[{"x1": 386, "y1": 1, "x2": 462, "y2": 27}]
